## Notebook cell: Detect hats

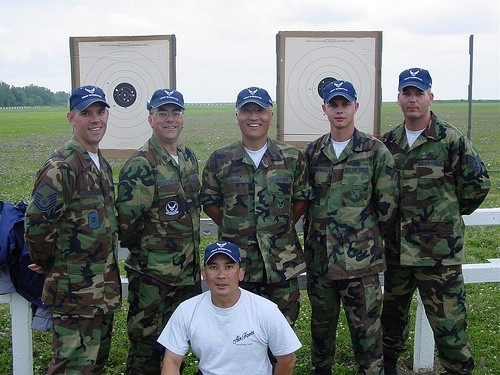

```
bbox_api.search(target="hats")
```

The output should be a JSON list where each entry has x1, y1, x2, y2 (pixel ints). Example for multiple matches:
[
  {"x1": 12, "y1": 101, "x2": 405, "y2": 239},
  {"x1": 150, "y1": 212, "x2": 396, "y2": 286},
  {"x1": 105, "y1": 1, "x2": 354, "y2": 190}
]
[
  {"x1": 398, "y1": 67, "x2": 432, "y2": 91},
  {"x1": 322, "y1": 80, "x2": 357, "y2": 104},
  {"x1": 69, "y1": 85, "x2": 110, "y2": 112},
  {"x1": 236, "y1": 87, "x2": 273, "y2": 109},
  {"x1": 204, "y1": 241, "x2": 241, "y2": 268},
  {"x1": 147, "y1": 89, "x2": 186, "y2": 110}
]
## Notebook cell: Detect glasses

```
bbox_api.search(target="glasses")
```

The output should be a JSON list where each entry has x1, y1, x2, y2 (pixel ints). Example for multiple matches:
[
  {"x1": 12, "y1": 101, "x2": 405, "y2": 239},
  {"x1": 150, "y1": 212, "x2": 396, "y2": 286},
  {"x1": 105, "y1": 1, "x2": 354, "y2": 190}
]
[{"x1": 151, "y1": 109, "x2": 184, "y2": 119}]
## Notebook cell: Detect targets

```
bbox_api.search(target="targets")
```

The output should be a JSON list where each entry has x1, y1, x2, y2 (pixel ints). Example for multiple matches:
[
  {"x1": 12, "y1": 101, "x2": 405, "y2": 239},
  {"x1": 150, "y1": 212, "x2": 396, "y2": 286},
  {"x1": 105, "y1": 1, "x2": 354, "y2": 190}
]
[
  {"x1": 287, "y1": 45, "x2": 374, "y2": 133},
  {"x1": 83, "y1": 49, "x2": 168, "y2": 141}
]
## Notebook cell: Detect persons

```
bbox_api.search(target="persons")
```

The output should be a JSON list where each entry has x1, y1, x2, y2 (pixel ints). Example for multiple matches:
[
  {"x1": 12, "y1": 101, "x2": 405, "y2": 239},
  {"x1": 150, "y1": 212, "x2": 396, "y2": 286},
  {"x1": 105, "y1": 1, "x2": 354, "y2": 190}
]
[
  {"x1": 115, "y1": 89, "x2": 203, "y2": 375},
  {"x1": 156, "y1": 241, "x2": 302, "y2": 375},
  {"x1": 302, "y1": 80, "x2": 398, "y2": 375},
  {"x1": 201, "y1": 87, "x2": 314, "y2": 375},
  {"x1": 381, "y1": 68, "x2": 491, "y2": 375},
  {"x1": 24, "y1": 85, "x2": 122, "y2": 375}
]
